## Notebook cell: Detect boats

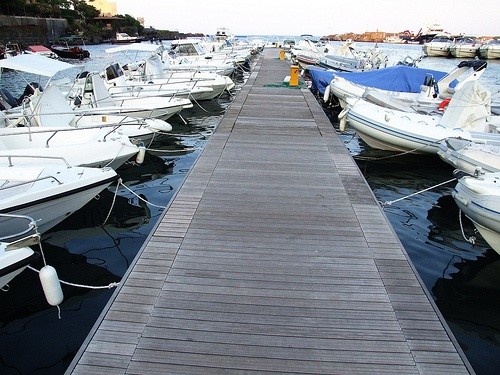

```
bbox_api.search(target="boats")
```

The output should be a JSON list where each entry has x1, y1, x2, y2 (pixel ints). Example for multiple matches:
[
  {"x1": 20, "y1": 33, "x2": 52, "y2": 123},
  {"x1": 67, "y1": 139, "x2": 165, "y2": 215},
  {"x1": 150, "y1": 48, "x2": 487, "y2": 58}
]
[
  {"x1": 479, "y1": 38, "x2": 500, "y2": 60},
  {"x1": 288, "y1": 34, "x2": 500, "y2": 156},
  {"x1": 0, "y1": 32, "x2": 277, "y2": 302},
  {"x1": 281, "y1": 40, "x2": 295, "y2": 48},
  {"x1": 449, "y1": 35, "x2": 480, "y2": 58},
  {"x1": 422, "y1": 34, "x2": 452, "y2": 56},
  {"x1": 451, "y1": 170, "x2": 500, "y2": 257},
  {"x1": 416, "y1": 22, "x2": 445, "y2": 41},
  {"x1": 436, "y1": 135, "x2": 500, "y2": 175}
]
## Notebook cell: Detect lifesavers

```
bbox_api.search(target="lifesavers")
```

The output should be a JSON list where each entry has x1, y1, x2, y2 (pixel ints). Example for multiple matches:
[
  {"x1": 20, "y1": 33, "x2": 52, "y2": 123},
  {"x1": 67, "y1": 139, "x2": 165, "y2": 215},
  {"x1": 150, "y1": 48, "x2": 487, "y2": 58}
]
[{"x1": 439, "y1": 98, "x2": 451, "y2": 112}]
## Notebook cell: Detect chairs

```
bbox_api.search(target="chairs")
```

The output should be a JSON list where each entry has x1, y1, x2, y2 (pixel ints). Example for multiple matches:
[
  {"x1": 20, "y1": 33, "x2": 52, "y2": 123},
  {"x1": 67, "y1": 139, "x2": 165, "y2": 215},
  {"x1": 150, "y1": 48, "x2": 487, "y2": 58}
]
[
  {"x1": 447, "y1": 88, "x2": 454, "y2": 94},
  {"x1": 0, "y1": 88, "x2": 16, "y2": 111},
  {"x1": 106, "y1": 63, "x2": 124, "y2": 80},
  {"x1": 77, "y1": 72, "x2": 89, "y2": 78}
]
